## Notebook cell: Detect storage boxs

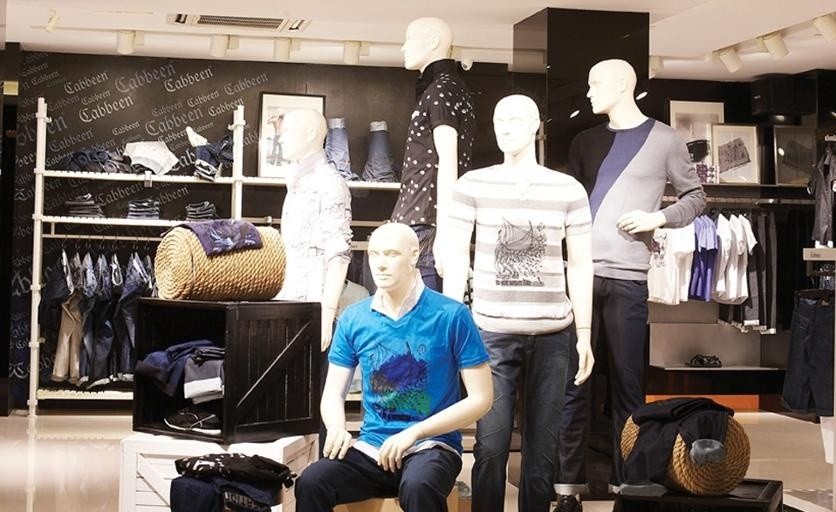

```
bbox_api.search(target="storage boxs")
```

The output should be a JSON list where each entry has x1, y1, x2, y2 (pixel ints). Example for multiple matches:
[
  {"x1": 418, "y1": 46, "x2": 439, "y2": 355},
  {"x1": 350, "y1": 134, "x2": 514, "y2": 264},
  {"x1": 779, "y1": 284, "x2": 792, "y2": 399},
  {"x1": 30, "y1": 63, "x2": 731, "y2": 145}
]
[
  {"x1": 118, "y1": 429, "x2": 325, "y2": 511},
  {"x1": 126, "y1": 293, "x2": 326, "y2": 446}
]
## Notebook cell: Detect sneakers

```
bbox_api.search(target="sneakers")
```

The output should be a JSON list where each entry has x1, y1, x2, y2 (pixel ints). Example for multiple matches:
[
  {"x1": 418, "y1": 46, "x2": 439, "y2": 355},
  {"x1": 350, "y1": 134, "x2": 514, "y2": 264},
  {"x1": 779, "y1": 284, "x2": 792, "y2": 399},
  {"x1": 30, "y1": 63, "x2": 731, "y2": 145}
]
[
  {"x1": 552, "y1": 495, "x2": 582, "y2": 512},
  {"x1": 163, "y1": 408, "x2": 222, "y2": 436}
]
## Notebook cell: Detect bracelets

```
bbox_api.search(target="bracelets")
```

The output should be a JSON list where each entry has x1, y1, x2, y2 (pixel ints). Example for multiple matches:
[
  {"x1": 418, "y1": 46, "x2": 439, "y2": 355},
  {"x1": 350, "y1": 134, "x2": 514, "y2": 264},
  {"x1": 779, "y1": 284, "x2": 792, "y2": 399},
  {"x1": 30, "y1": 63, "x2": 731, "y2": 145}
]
[{"x1": 574, "y1": 324, "x2": 592, "y2": 332}]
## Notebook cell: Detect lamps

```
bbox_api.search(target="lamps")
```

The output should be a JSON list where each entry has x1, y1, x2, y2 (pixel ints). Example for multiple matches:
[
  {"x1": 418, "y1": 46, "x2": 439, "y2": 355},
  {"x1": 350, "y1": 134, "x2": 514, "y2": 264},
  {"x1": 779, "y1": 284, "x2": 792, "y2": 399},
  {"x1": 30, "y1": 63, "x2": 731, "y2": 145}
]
[
  {"x1": 113, "y1": 26, "x2": 362, "y2": 66},
  {"x1": 712, "y1": 11, "x2": 836, "y2": 72}
]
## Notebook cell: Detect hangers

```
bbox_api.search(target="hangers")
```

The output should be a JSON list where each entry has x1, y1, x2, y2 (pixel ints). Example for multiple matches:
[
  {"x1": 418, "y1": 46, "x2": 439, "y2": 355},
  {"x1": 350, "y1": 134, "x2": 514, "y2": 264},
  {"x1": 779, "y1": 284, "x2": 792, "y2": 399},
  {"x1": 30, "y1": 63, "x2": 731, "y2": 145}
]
[
  {"x1": 823, "y1": 136, "x2": 832, "y2": 154},
  {"x1": 697, "y1": 202, "x2": 774, "y2": 220},
  {"x1": 48, "y1": 230, "x2": 164, "y2": 259},
  {"x1": 788, "y1": 269, "x2": 836, "y2": 301}
]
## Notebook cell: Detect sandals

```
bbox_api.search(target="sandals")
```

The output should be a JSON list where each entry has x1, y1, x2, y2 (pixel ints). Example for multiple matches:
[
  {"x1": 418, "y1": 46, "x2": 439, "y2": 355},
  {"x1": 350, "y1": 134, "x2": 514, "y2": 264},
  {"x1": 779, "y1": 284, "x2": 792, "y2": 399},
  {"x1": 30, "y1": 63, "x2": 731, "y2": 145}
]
[{"x1": 685, "y1": 354, "x2": 722, "y2": 368}]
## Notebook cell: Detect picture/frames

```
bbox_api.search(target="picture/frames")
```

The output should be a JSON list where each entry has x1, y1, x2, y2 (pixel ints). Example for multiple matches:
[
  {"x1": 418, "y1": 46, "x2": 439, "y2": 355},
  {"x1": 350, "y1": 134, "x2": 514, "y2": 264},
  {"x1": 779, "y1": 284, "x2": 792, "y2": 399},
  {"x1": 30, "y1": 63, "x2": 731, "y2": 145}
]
[
  {"x1": 771, "y1": 122, "x2": 823, "y2": 191},
  {"x1": 709, "y1": 122, "x2": 763, "y2": 188},
  {"x1": 662, "y1": 94, "x2": 728, "y2": 184},
  {"x1": 255, "y1": 87, "x2": 327, "y2": 181}
]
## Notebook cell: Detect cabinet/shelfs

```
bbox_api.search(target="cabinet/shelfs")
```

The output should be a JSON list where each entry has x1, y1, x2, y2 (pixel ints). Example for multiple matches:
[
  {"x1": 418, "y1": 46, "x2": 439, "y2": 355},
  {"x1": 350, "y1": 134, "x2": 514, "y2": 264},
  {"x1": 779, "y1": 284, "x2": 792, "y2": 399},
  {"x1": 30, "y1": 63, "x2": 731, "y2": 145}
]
[{"x1": 26, "y1": 92, "x2": 408, "y2": 433}]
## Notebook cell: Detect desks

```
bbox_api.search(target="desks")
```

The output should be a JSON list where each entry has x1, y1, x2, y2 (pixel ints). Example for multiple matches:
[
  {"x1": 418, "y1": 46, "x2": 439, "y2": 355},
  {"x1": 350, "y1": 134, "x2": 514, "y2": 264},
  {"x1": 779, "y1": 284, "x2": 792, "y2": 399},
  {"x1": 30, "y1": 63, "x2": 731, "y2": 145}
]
[{"x1": 610, "y1": 471, "x2": 785, "y2": 512}]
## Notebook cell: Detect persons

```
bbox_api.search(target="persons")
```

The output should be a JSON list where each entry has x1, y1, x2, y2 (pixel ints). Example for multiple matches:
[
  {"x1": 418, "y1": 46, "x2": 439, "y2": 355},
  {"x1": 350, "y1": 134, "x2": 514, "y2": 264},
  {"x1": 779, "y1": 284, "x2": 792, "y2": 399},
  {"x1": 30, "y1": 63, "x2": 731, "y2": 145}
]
[
  {"x1": 295, "y1": 226, "x2": 496, "y2": 512},
  {"x1": 441, "y1": 95, "x2": 596, "y2": 511},
  {"x1": 389, "y1": 17, "x2": 477, "y2": 293},
  {"x1": 551, "y1": 59, "x2": 709, "y2": 512},
  {"x1": 270, "y1": 106, "x2": 354, "y2": 393}
]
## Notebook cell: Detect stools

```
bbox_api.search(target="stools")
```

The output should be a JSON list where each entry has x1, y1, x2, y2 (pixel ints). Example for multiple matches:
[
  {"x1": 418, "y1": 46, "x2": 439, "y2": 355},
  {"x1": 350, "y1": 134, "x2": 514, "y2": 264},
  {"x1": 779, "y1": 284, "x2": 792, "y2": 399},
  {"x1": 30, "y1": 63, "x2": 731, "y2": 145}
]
[{"x1": 332, "y1": 486, "x2": 459, "y2": 512}]
